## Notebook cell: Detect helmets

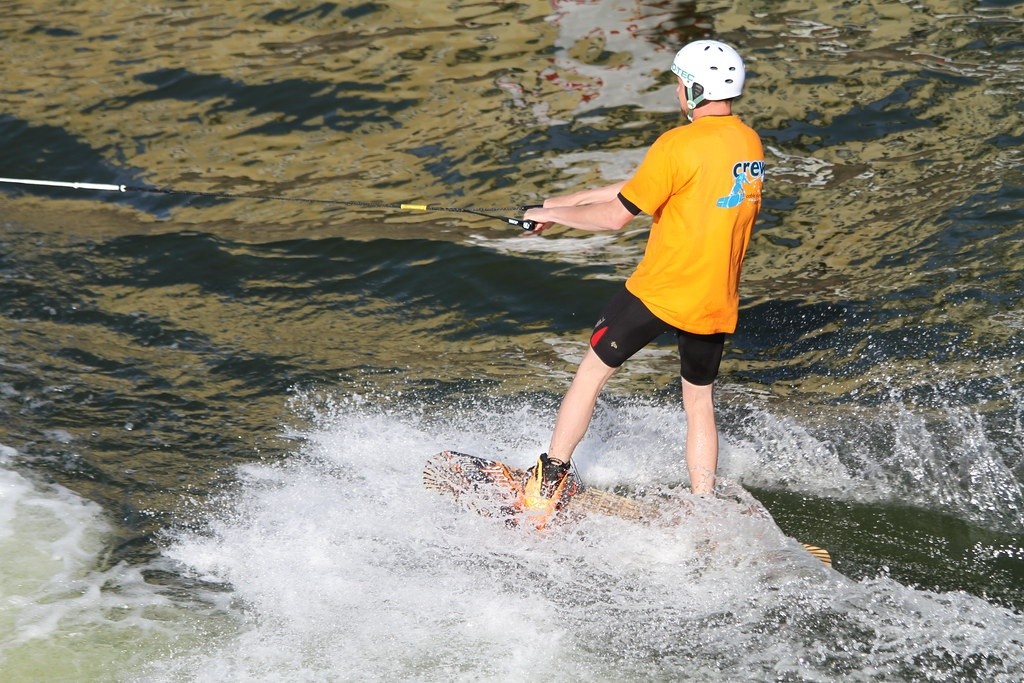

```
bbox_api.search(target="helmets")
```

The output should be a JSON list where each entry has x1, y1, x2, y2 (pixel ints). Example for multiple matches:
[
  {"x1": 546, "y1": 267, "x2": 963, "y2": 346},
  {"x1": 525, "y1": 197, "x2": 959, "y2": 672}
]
[{"x1": 671, "y1": 39, "x2": 746, "y2": 100}]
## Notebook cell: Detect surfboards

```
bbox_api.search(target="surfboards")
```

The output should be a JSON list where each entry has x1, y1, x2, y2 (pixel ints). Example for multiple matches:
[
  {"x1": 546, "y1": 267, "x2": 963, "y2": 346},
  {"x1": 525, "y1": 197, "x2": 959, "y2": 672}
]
[{"x1": 422, "y1": 450, "x2": 831, "y2": 595}]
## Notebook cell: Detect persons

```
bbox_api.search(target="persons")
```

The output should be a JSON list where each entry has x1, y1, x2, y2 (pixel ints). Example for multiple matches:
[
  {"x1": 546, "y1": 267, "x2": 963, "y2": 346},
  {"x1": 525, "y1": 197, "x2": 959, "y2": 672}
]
[{"x1": 524, "y1": 40, "x2": 765, "y2": 530}]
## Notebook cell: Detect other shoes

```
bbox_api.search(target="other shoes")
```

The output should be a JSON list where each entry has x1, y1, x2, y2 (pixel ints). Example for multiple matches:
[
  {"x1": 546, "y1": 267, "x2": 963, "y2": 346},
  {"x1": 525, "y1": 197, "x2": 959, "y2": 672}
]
[{"x1": 522, "y1": 453, "x2": 579, "y2": 511}]
